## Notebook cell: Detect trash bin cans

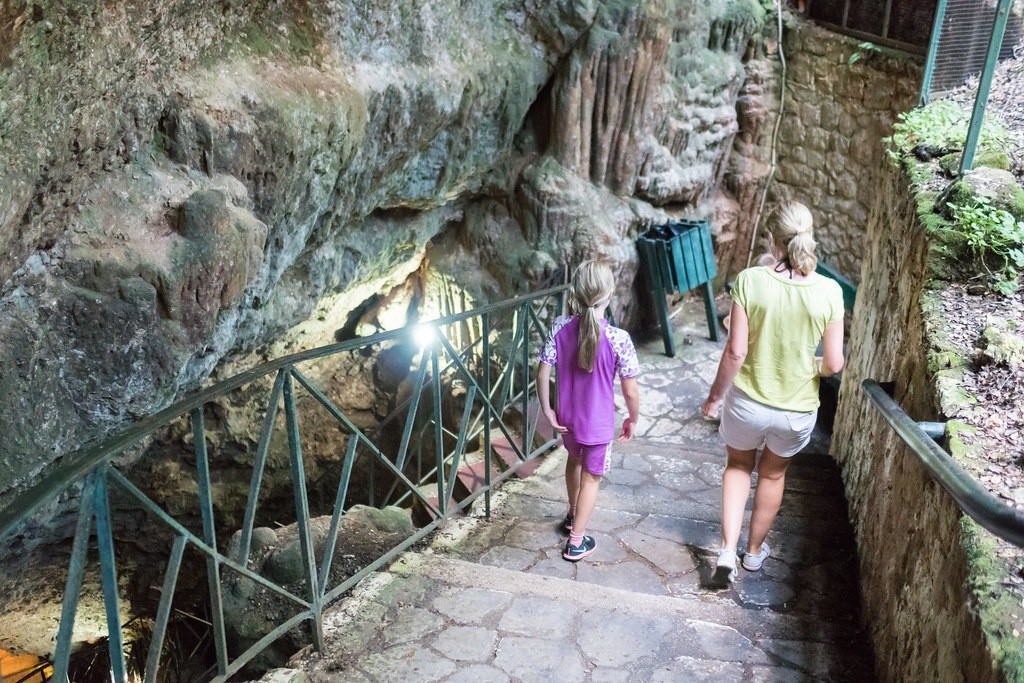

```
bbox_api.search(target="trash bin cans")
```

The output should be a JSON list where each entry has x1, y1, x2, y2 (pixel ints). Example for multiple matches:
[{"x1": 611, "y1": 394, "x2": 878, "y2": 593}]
[{"x1": 639, "y1": 219, "x2": 720, "y2": 293}]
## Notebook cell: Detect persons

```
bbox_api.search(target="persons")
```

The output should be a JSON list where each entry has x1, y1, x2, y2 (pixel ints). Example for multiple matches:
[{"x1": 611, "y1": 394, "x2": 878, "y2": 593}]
[
  {"x1": 536, "y1": 259, "x2": 643, "y2": 561},
  {"x1": 699, "y1": 200, "x2": 844, "y2": 584}
]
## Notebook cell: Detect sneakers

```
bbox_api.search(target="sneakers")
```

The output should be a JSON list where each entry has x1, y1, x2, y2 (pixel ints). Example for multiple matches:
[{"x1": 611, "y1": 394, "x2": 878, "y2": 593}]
[
  {"x1": 712, "y1": 550, "x2": 739, "y2": 584},
  {"x1": 563, "y1": 536, "x2": 596, "y2": 561},
  {"x1": 742, "y1": 541, "x2": 770, "y2": 570},
  {"x1": 564, "y1": 513, "x2": 573, "y2": 529}
]
